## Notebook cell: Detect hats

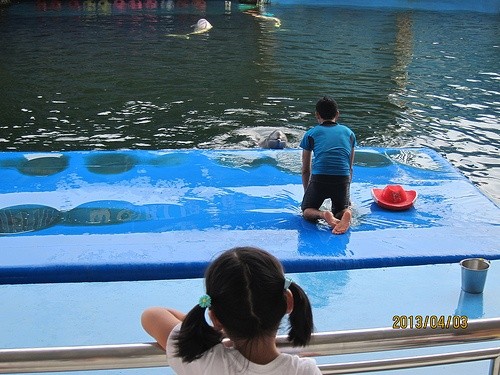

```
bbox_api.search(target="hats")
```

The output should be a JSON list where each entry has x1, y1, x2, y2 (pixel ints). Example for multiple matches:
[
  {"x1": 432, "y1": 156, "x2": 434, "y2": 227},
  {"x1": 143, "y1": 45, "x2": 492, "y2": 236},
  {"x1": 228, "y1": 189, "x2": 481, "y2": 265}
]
[{"x1": 371, "y1": 185, "x2": 417, "y2": 209}]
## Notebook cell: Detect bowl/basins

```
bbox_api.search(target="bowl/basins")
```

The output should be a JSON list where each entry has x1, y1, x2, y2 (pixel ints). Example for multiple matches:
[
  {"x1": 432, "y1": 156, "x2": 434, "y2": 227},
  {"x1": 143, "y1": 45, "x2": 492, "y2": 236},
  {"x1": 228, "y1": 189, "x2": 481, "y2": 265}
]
[{"x1": 459, "y1": 258, "x2": 490, "y2": 295}]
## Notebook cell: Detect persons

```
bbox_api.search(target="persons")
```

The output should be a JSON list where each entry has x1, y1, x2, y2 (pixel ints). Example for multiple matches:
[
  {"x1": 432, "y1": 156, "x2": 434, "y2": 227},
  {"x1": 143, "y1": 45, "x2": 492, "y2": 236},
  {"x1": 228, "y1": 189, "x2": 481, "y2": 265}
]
[
  {"x1": 301, "y1": 97, "x2": 357, "y2": 235},
  {"x1": 141, "y1": 246, "x2": 321, "y2": 375}
]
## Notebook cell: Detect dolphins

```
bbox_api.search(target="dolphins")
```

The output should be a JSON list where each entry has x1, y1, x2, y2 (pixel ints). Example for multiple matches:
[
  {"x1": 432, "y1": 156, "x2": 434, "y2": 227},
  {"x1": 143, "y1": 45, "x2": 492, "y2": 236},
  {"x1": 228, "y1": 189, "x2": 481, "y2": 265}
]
[
  {"x1": 259, "y1": 129, "x2": 287, "y2": 149},
  {"x1": 165, "y1": 18, "x2": 213, "y2": 39},
  {"x1": 243, "y1": 12, "x2": 281, "y2": 28}
]
